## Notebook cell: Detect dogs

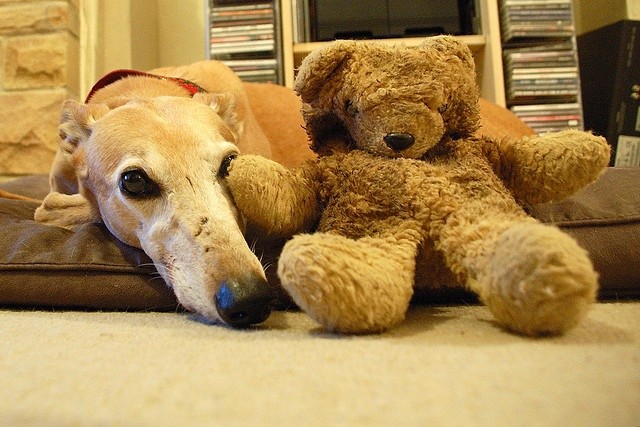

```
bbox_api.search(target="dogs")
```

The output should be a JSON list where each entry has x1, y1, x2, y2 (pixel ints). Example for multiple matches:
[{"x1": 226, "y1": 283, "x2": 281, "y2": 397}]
[{"x1": 32, "y1": 59, "x2": 539, "y2": 329}]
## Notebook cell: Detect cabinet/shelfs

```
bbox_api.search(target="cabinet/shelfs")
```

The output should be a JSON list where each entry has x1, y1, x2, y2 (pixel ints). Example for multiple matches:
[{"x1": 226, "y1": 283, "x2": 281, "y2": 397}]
[{"x1": 280, "y1": 0, "x2": 506, "y2": 113}]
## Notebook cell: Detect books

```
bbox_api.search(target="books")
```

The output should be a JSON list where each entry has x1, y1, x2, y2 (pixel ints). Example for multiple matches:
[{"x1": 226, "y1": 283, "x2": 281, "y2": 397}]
[
  {"x1": 576, "y1": 20, "x2": 640, "y2": 168},
  {"x1": 499, "y1": 0, "x2": 576, "y2": 42},
  {"x1": 509, "y1": 104, "x2": 581, "y2": 136},
  {"x1": 208, "y1": 0, "x2": 277, "y2": 84},
  {"x1": 501, "y1": 43, "x2": 579, "y2": 100}
]
